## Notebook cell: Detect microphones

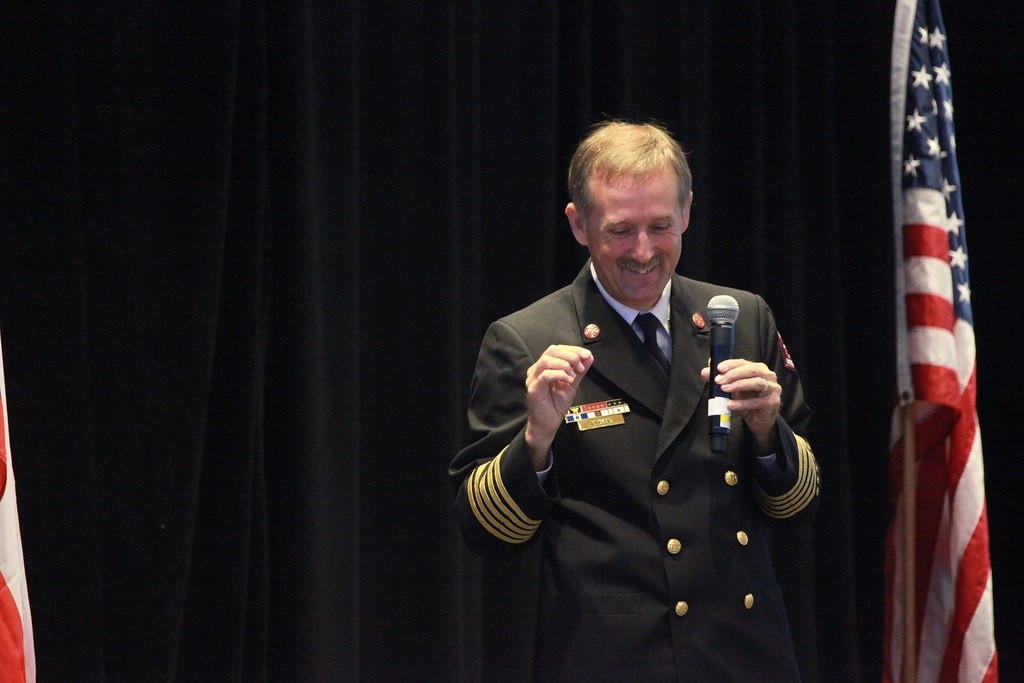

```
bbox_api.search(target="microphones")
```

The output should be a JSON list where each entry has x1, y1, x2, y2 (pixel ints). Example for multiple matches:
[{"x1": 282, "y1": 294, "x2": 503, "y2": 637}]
[{"x1": 705, "y1": 294, "x2": 739, "y2": 452}]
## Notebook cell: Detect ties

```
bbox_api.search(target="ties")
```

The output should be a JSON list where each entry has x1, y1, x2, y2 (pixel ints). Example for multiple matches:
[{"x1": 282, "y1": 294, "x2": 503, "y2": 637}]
[{"x1": 636, "y1": 312, "x2": 671, "y2": 378}]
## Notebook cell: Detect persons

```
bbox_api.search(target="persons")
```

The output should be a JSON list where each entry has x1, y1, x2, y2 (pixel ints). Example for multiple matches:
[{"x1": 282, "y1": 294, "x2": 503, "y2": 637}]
[{"x1": 448, "y1": 123, "x2": 821, "y2": 683}]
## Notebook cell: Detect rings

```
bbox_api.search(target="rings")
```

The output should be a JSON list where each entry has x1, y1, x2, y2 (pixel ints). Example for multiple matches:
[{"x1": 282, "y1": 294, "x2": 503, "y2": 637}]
[{"x1": 760, "y1": 379, "x2": 767, "y2": 392}]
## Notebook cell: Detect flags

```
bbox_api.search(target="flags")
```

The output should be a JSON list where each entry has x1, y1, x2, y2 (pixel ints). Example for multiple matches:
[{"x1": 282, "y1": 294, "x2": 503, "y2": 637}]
[
  {"x1": 0, "y1": 335, "x2": 37, "y2": 683},
  {"x1": 882, "y1": 0, "x2": 997, "y2": 683}
]
